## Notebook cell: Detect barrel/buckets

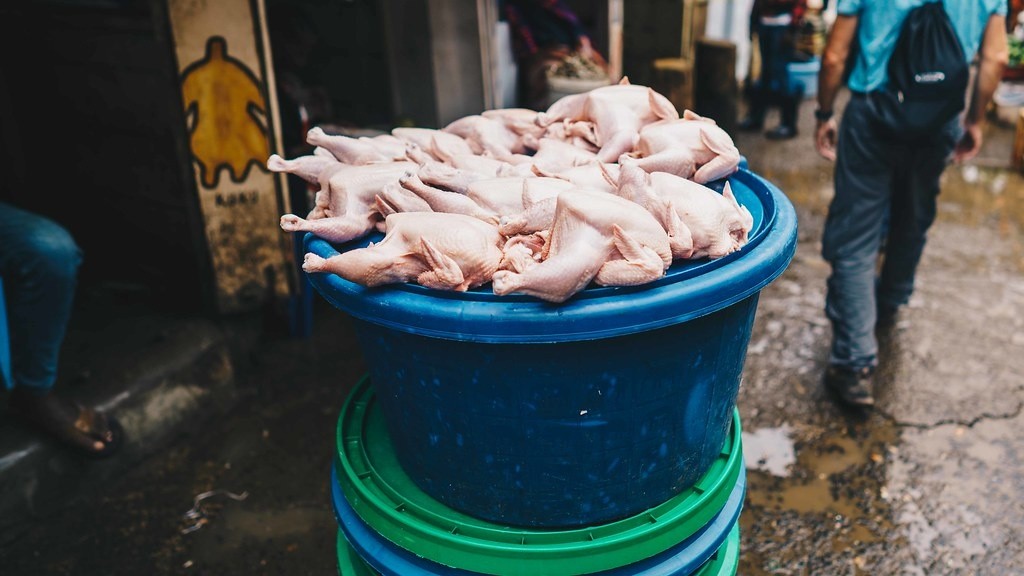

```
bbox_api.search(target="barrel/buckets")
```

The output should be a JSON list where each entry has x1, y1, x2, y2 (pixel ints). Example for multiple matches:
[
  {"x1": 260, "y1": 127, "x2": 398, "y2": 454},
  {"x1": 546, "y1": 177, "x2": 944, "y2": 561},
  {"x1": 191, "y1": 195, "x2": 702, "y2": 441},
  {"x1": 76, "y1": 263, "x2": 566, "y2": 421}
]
[{"x1": 301, "y1": 158, "x2": 798, "y2": 575}]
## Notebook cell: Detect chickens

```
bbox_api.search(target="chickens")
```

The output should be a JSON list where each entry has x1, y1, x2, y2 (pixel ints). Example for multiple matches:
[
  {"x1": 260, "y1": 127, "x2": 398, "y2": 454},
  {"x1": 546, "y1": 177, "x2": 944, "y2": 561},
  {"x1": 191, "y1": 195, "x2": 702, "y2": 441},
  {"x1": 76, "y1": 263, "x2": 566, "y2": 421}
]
[
  {"x1": 492, "y1": 181, "x2": 673, "y2": 303},
  {"x1": 267, "y1": 76, "x2": 679, "y2": 243},
  {"x1": 301, "y1": 212, "x2": 511, "y2": 292},
  {"x1": 619, "y1": 88, "x2": 740, "y2": 185},
  {"x1": 616, "y1": 155, "x2": 754, "y2": 260}
]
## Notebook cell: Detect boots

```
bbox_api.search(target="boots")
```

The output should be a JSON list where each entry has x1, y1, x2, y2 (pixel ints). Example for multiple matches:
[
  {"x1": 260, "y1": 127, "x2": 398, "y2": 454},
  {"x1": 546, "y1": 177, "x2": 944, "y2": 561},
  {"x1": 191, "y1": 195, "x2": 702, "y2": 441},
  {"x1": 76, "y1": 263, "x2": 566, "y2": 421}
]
[
  {"x1": 765, "y1": 109, "x2": 799, "y2": 140},
  {"x1": 736, "y1": 99, "x2": 765, "y2": 133}
]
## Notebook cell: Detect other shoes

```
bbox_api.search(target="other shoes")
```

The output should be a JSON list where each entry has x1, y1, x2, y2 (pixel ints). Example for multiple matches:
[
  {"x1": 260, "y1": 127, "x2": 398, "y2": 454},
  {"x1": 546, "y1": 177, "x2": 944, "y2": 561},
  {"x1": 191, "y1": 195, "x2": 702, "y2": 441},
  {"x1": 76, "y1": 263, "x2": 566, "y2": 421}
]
[
  {"x1": 877, "y1": 320, "x2": 901, "y2": 341},
  {"x1": 821, "y1": 362, "x2": 875, "y2": 409}
]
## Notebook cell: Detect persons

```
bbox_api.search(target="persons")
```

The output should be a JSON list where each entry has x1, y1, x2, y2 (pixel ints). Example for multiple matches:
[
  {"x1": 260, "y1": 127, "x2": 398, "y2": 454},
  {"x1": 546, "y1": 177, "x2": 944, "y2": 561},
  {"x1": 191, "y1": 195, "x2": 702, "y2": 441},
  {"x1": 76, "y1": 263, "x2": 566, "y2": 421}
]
[
  {"x1": 1, "y1": 203, "x2": 126, "y2": 460},
  {"x1": 504, "y1": 1, "x2": 594, "y2": 112},
  {"x1": 739, "y1": 0, "x2": 828, "y2": 140},
  {"x1": 814, "y1": 0, "x2": 1010, "y2": 419}
]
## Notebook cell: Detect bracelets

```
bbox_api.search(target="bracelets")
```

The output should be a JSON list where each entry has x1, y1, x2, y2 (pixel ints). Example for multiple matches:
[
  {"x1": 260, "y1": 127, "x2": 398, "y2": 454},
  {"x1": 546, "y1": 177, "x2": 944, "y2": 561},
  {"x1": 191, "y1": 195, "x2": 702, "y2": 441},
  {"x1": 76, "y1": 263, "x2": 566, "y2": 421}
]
[{"x1": 815, "y1": 109, "x2": 833, "y2": 121}]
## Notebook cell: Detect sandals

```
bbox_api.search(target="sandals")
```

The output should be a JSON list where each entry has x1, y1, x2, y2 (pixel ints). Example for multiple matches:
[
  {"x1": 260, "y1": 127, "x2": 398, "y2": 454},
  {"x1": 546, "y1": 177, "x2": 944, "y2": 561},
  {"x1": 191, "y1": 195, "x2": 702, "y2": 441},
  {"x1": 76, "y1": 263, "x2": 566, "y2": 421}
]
[{"x1": 7, "y1": 387, "x2": 123, "y2": 460}]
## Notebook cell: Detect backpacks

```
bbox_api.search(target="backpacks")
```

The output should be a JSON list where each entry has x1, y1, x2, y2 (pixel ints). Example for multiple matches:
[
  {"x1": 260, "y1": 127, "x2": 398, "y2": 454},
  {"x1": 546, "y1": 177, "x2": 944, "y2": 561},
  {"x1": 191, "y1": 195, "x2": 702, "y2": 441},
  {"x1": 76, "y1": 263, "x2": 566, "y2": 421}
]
[{"x1": 867, "y1": 1, "x2": 969, "y2": 146}]
